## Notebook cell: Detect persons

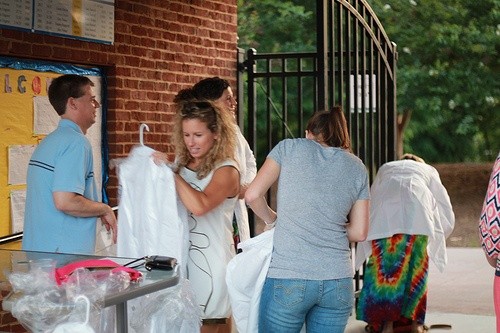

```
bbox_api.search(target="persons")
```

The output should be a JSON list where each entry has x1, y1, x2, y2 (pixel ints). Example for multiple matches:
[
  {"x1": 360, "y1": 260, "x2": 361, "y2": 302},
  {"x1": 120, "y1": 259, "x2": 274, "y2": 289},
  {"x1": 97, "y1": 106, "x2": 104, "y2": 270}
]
[
  {"x1": 358, "y1": 154, "x2": 455, "y2": 333},
  {"x1": 21, "y1": 74, "x2": 118, "y2": 275},
  {"x1": 244, "y1": 106, "x2": 371, "y2": 332},
  {"x1": 478, "y1": 151, "x2": 500, "y2": 333},
  {"x1": 129, "y1": 77, "x2": 257, "y2": 333}
]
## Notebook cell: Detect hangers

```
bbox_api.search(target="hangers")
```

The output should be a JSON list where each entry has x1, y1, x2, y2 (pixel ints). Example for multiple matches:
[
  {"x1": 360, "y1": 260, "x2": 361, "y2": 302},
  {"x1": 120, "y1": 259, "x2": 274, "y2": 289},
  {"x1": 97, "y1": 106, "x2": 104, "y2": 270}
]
[{"x1": 139, "y1": 124, "x2": 149, "y2": 147}]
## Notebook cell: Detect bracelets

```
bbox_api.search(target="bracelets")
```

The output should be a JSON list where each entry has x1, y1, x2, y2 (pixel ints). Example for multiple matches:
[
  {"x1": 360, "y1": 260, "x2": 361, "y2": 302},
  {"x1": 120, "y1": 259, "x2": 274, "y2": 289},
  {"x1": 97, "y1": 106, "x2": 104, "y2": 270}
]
[{"x1": 264, "y1": 218, "x2": 277, "y2": 228}]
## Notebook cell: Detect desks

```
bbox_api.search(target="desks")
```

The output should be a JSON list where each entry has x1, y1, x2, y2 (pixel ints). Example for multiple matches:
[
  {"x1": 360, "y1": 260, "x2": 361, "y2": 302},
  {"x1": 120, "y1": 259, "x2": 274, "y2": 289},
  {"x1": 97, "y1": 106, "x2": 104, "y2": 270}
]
[{"x1": 0, "y1": 248, "x2": 181, "y2": 333}]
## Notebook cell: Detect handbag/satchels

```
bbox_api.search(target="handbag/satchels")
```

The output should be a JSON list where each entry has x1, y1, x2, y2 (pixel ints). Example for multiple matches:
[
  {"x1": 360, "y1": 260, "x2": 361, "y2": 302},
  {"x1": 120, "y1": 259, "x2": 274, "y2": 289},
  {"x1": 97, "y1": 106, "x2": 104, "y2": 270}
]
[
  {"x1": 53, "y1": 259, "x2": 142, "y2": 287},
  {"x1": 145, "y1": 255, "x2": 178, "y2": 271}
]
